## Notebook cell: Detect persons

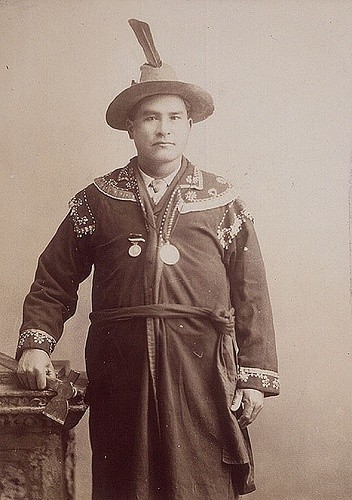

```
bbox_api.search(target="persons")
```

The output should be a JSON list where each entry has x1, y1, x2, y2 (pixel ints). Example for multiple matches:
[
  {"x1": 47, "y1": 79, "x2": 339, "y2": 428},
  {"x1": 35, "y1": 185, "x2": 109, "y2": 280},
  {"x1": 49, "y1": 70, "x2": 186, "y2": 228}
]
[{"x1": 15, "y1": 60, "x2": 280, "y2": 500}]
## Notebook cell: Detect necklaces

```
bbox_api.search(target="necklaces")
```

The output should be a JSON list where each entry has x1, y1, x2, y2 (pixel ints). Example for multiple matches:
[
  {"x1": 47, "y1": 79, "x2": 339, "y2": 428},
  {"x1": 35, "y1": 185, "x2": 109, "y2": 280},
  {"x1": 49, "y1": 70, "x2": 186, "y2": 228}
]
[{"x1": 127, "y1": 162, "x2": 195, "y2": 265}]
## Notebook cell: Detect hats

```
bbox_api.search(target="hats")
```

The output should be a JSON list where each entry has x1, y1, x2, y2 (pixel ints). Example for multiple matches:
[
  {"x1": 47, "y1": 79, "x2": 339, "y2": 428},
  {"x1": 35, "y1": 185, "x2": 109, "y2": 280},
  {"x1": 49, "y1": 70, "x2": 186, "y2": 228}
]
[{"x1": 106, "y1": 19, "x2": 214, "y2": 131}]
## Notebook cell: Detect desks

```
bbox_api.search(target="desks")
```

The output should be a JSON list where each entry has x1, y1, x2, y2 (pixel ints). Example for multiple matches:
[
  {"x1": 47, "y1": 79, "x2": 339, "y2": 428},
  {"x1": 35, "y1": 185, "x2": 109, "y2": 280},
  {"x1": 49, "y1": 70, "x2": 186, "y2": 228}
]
[{"x1": 0, "y1": 360, "x2": 88, "y2": 500}]
